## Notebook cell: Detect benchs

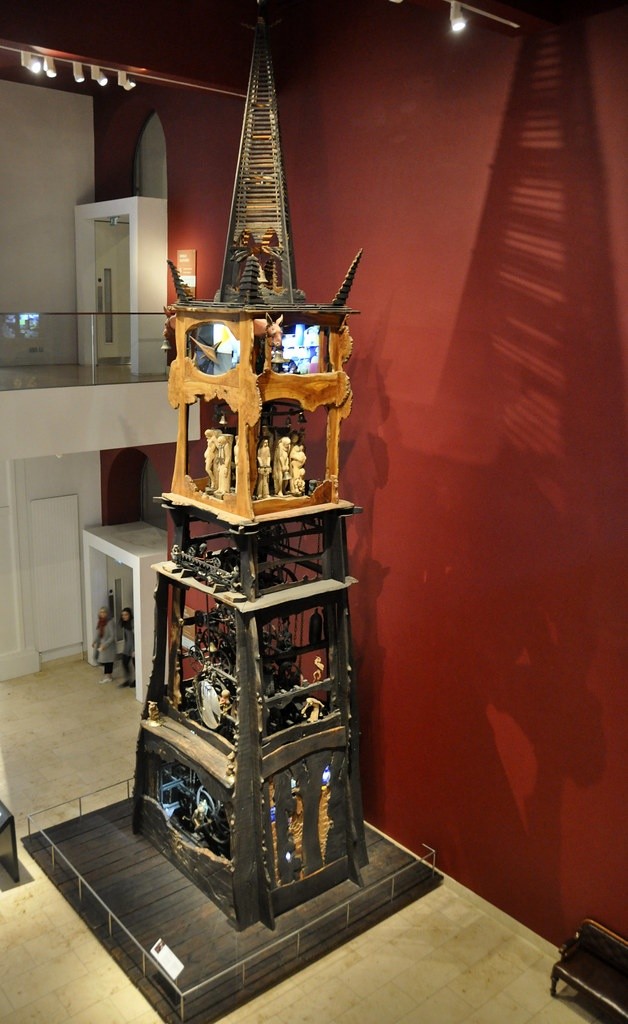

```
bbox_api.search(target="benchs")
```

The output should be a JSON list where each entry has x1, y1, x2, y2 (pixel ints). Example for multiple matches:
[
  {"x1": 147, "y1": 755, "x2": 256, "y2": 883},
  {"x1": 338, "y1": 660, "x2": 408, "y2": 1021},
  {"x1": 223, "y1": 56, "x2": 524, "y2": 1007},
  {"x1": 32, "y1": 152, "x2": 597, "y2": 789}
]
[{"x1": 549, "y1": 917, "x2": 628, "y2": 1024}]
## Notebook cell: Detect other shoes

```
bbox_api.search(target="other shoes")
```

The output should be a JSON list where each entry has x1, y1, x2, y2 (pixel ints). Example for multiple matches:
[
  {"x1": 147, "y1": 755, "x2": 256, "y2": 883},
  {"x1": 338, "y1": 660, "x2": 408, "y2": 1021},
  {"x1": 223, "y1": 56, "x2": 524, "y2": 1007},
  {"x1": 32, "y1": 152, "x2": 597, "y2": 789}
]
[
  {"x1": 100, "y1": 678, "x2": 112, "y2": 683},
  {"x1": 123, "y1": 681, "x2": 130, "y2": 686},
  {"x1": 130, "y1": 680, "x2": 135, "y2": 687}
]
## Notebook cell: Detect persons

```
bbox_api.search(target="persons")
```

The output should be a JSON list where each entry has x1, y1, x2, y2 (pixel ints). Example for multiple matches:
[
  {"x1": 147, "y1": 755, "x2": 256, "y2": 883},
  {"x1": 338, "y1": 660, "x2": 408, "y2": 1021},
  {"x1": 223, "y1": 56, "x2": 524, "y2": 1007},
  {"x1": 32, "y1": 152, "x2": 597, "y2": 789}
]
[
  {"x1": 92, "y1": 606, "x2": 118, "y2": 685},
  {"x1": 203, "y1": 426, "x2": 307, "y2": 499},
  {"x1": 118, "y1": 607, "x2": 136, "y2": 689}
]
